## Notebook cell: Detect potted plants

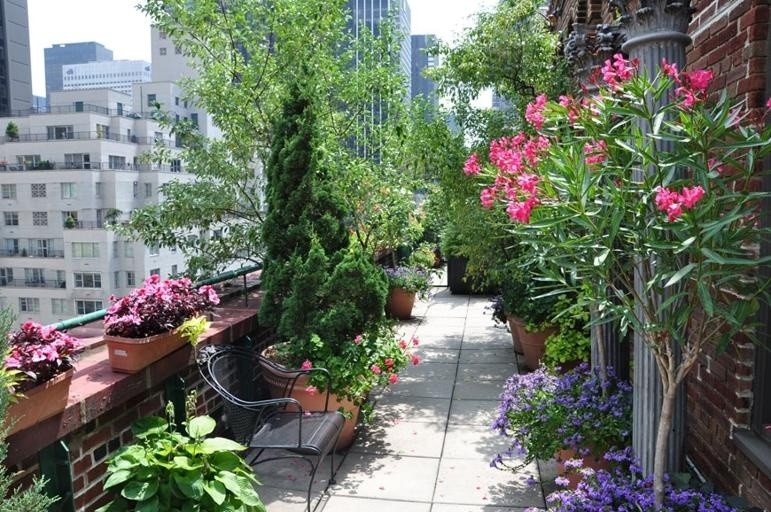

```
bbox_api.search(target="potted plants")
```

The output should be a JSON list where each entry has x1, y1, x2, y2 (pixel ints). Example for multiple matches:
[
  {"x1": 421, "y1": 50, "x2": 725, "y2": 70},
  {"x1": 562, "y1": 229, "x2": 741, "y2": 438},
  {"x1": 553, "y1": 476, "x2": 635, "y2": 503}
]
[
  {"x1": 105, "y1": 0, "x2": 418, "y2": 455},
  {"x1": 486, "y1": 289, "x2": 524, "y2": 355},
  {"x1": 0, "y1": 304, "x2": 62, "y2": 512},
  {"x1": 500, "y1": 259, "x2": 560, "y2": 369},
  {"x1": 102, "y1": 389, "x2": 267, "y2": 512}
]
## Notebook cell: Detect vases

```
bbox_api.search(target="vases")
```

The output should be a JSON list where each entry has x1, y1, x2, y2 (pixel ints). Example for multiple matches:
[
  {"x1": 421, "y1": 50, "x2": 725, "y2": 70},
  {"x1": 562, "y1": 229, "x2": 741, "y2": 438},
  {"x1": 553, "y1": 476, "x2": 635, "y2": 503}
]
[
  {"x1": 5, "y1": 369, "x2": 73, "y2": 437},
  {"x1": 386, "y1": 287, "x2": 415, "y2": 319},
  {"x1": 105, "y1": 329, "x2": 191, "y2": 375}
]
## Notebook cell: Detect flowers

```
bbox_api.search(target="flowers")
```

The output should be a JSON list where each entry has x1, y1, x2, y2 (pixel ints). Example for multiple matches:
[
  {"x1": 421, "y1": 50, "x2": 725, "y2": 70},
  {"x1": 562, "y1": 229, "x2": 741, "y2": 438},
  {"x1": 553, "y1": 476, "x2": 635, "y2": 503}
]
[
  {"x1": 490, "y1": 361, "x2": 632, "y2": 472},
  {"x1": 528, "y1": 447, "x2": 739, "y2": 512},
  {"x1": 299, "y1": 329, "x2": 420, "y2": 424},
  {"x1": 6, "y1": 321, "x2": 83, "y2": 388},
  {"x1": 174, "y1": 314, "x2": 206, "y2": 359},
  {"x1": 461, "y1": 53, "x2": 771, "y2": 512},
  {"x1": 383, "y1": 265, "x2": 443, "y2": 302},
  {"x1": 102, "y1": 273, "x2": 219, "y2": 339}
]
